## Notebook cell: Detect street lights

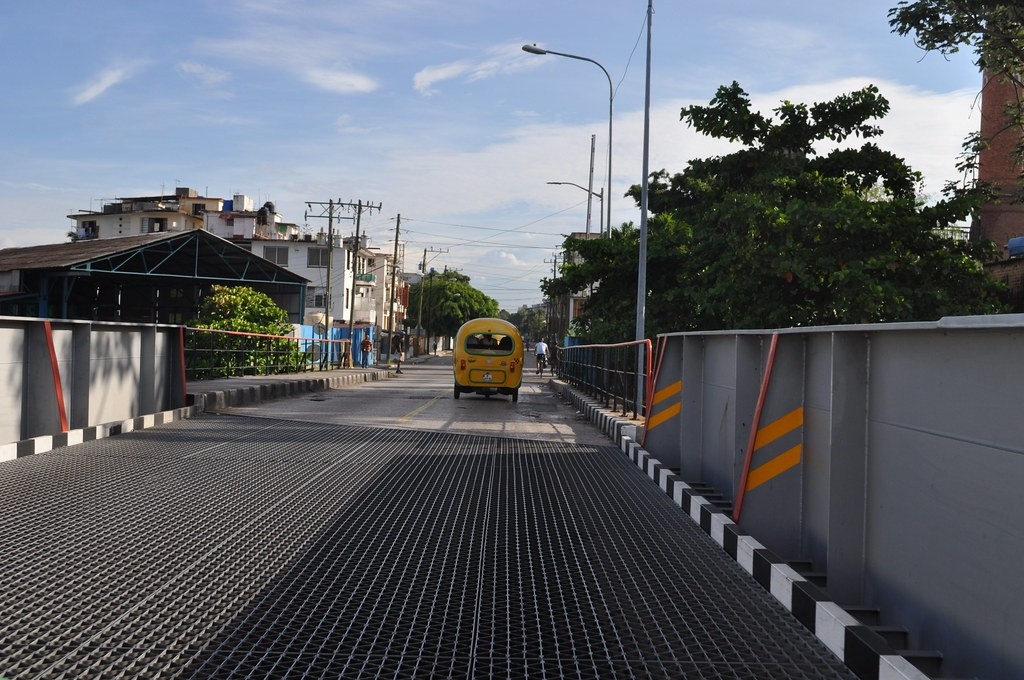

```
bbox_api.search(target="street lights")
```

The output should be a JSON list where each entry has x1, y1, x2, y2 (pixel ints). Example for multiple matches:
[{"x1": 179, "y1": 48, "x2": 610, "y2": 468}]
[
  {"x1": 522, "y1": 43, "x2": 614, "y2": 237},
  {"x1": 543, "y1": 181, "x2": 604, "y2": 237}
]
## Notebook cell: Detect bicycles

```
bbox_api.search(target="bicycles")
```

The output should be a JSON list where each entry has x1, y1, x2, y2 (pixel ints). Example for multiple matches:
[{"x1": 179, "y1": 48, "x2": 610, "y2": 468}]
[{"x1": 534, "y1": 352, "x2": 550, "y2": 377}]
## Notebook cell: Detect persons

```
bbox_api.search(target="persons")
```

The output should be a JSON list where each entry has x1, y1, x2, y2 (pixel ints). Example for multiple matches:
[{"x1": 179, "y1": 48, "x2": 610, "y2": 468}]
[
  {"x1": 467, "y1": 334, "x2": 513, "y2": 345},
  {"x1": 432, "y1": 341, "x2": 437, "y2": 354},
  {"x1": 361, "y1": 334, "x2": 372, "y2": 368},
  {"x1": 394, "y1": 334, "x2": 405, "y2": 374},
  {"x1": 534, "y1": 338, "x2": 549, "y2": 374}
]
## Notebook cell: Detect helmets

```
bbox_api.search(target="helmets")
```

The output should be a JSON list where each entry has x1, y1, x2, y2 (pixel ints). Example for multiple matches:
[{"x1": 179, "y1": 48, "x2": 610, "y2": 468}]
[{"x1": 400, "y1": 331, "x2": 406, "y2": 335}]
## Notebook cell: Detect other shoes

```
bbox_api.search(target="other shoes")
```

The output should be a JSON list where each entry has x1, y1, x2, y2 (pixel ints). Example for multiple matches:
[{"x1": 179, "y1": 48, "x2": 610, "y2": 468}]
[
  {"x1": 536, "y1": 370, "x2": 539, "y2": 374},
  {"x1": 396, "y1": 370, "x2": 403, "y2": 374}
]
